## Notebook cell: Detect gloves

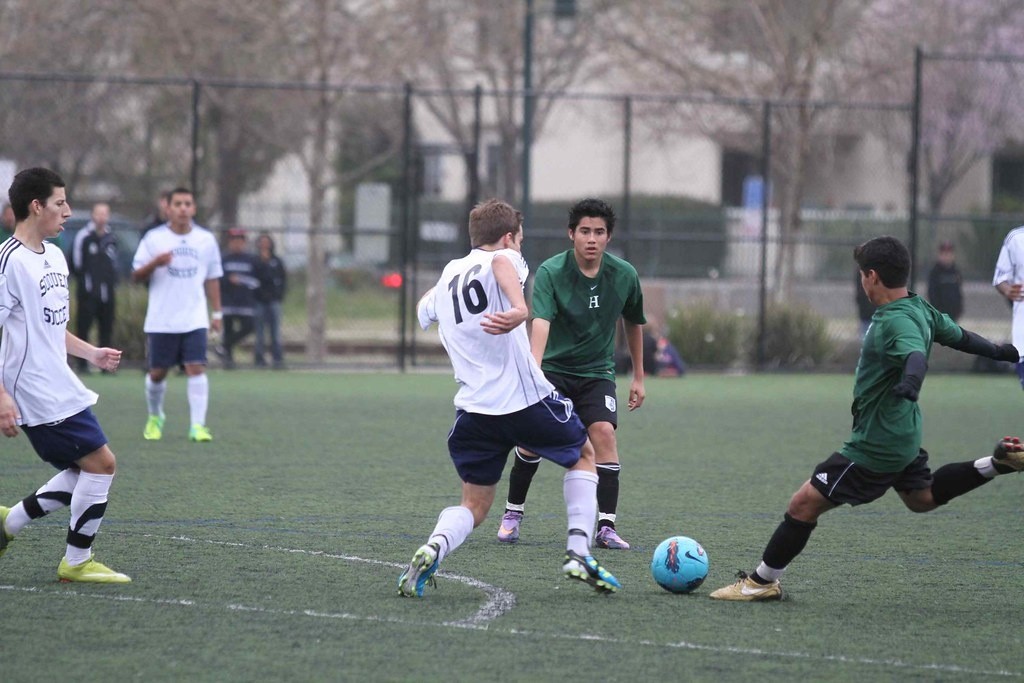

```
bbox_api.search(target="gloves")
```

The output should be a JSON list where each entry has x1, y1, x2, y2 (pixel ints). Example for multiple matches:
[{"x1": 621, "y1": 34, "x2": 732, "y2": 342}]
[
  {"x1": 954, "y1": 324, "x2": 1020, "y2": 364},
  {"x1": 894, "y1": 350, "x2": 928, "y2": 401}
]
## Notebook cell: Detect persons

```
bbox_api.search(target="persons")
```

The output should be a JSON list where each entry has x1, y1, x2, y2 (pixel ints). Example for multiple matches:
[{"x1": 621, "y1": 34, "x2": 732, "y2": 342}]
[
  {"x1": 929, "y1": 244, "x2": 963, "y2": 372},
  {"x1": 991, "y1": 226, "x2": 1024, "y2": 391},
  {"x1": 710, "y1": 236, "x2": 1024, "y2": 603},
  {"x1": 0, "y1": 202, "x2": 120, "y2": 377},
  {"x1": 0, "y1": 168, "x2": 133, "y2": 584},
  {"x1": 856, "y1": 267, "x2": 878, "y2": 337},
  {"x1": 397, "y1": 199, "x2": 622, "y2": 598},
  {"x1": 137, "y1": 191, "x2": 286, "y2": 376},
  {"x1": 130, "y1": 188, "x2": 224, "y2": 443},
  {"x1": 497, "y1": 199, "x2": 647, "y2": 549}
]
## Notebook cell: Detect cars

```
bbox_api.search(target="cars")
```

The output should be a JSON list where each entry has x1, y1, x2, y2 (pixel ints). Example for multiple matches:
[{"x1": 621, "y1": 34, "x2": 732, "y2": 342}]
[{"x1": 55, "y1": 211, "x2": 150, "y2": 278}]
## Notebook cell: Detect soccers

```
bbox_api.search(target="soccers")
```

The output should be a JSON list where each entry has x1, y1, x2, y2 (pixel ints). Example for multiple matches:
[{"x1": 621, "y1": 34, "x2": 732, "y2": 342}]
[{"x1": 651, "y1": 537, "x2": 709, "y2": 593}]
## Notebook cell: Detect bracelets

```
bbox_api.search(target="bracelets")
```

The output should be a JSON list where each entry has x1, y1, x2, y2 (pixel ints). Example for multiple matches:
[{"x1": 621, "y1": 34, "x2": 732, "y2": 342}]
[{"x1": 212, "y1": 312, "x2": 223, "y2": 319}]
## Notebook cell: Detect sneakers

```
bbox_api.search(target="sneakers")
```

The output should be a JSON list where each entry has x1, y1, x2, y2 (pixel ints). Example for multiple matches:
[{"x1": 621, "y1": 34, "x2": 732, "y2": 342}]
[
  {"x1": 188, "y1": 424, "x2": 213, "y2": 442},
  {"x1": 497, "y1": 510, "x2": 524, "y2": 542},
  {"x1": 397, "y1": 541, "x2": 440, "y2": 600},
  {"x1": 991, "y1": 435, "x2": 1024, "y2": 475},
  {"x1": 0, "y1": 506, "x2": 15, "y2": 553},
  {"x1": 56, "y1": 554, "x2": 133, "y2": 584},
  {"x1": 562, "y1": 549, "x2": 621, "y2": 595},
  {"x1": 595, "y1": 526, "x2": 629, "y2": 550},
  {"x1": 143, "y1": 414, "x2": 165, "y2": 440},
  {"x1": 710, "y1": 568, "x2": 784, "y2": 601}
]
